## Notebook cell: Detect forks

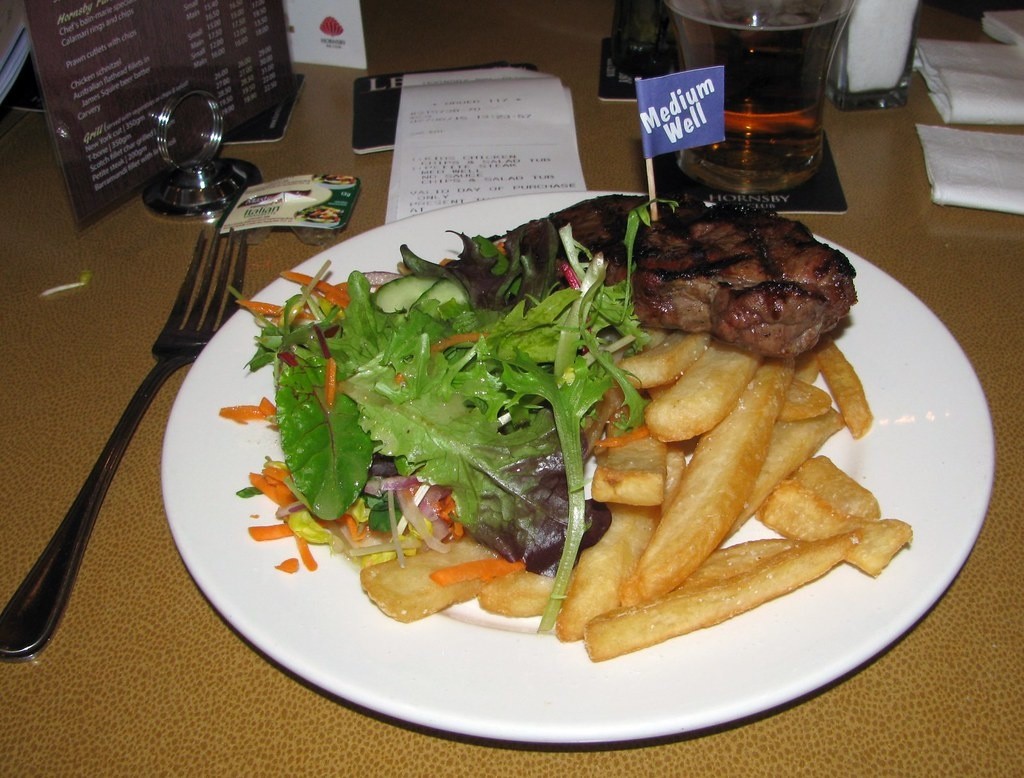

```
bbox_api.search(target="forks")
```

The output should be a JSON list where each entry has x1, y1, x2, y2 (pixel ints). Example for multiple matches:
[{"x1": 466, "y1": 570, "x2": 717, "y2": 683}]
[{"x1": 0, "y1": 224, "x2": 250, "y2": 663}]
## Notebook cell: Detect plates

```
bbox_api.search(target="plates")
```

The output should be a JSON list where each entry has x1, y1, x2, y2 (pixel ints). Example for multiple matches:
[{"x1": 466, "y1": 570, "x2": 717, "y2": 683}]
[{"x1": 155, "y1": 189, "x2": 1000, "y2": 745}]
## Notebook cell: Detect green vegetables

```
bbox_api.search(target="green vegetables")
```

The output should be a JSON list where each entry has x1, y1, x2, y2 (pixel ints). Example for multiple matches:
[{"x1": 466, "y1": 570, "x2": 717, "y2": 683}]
[{"x1": 268, "y1": 199, "x2": 681, "y2": 636}]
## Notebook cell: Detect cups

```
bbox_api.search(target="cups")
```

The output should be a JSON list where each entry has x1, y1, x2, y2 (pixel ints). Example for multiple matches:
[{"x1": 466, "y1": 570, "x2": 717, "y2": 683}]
[
  {"x1": 826, "y1": 0, "x2": 922, "y2": 110},
  {"x1": 611, "y1": 0, "x2": 677, "y2": 79},
  {"x1": 662, "y1": 0, "x2": 860, "y2": 193}
]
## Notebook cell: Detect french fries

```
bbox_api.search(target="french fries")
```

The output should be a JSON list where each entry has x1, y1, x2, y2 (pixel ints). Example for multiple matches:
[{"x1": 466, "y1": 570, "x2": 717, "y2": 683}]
[{"x1": 361, "y1": 329, "x2": 912, "y2": 661}]
